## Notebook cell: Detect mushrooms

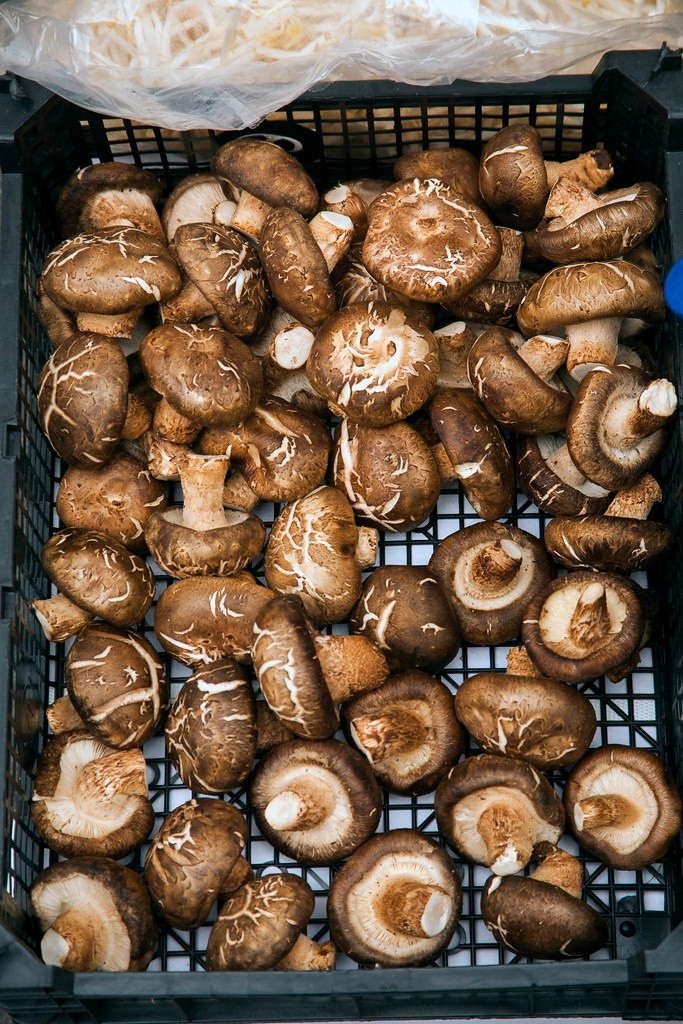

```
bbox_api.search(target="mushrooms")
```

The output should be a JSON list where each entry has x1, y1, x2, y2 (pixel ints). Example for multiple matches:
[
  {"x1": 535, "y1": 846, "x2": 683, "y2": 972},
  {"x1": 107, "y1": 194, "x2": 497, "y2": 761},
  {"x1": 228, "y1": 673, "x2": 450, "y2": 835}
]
[{"x1": 28, "y1": 121, "x2": 678, "y2": 974}]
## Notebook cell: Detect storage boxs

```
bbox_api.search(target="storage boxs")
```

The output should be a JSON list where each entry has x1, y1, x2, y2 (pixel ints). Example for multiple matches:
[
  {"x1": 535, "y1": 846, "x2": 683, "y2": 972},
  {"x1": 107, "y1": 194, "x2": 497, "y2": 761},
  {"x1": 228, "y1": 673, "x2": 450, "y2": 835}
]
[{"x1": 0, "y1": 41, "x2": 683, "y2": 1023}]
